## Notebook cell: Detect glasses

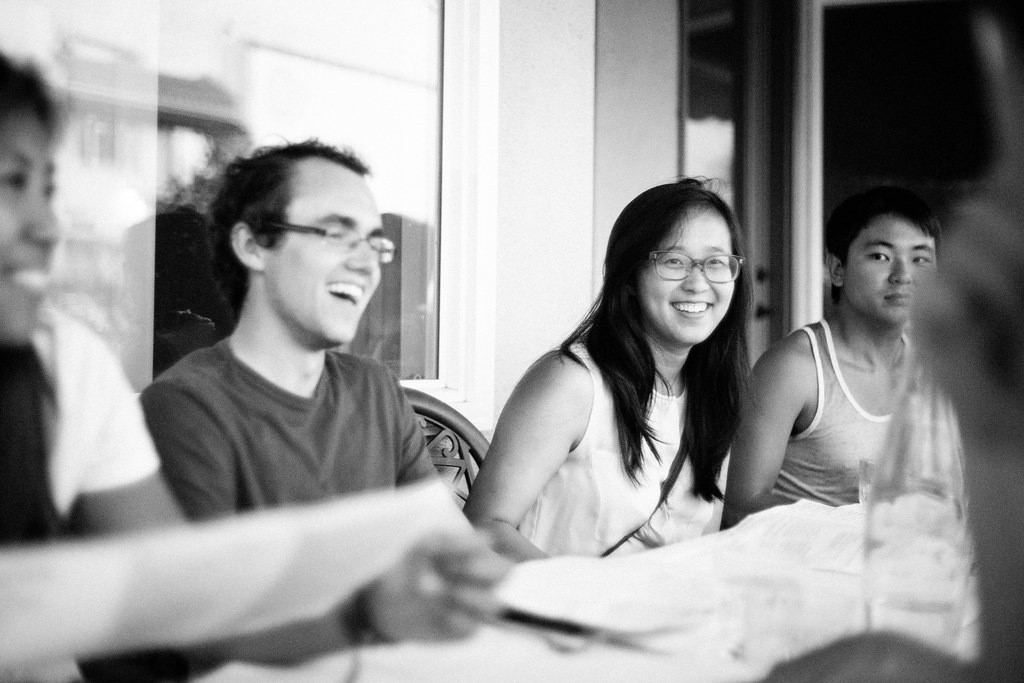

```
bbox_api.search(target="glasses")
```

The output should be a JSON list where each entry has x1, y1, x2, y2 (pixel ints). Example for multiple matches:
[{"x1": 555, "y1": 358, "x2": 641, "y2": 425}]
[
  {"x1": 248, "y1": 219, "x2": 399, "y2": 267},
  {"x1": 638, "y1": 249, "x2": 746, "y2": 284}
]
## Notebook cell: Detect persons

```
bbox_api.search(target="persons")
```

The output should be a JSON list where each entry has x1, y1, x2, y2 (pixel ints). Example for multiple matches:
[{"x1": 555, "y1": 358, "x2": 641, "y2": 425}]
[
  {"x1": 141, "y1": 140, "x2": 433, "y2": 524},
  {"x1": 767, "y1": 148, "x2": 1024, "y2": 683},
  {"x1": 0, "y1": 52, "x2": 508, "y2": 683},
  {"x1": 461, "y1": 179, "x2": 751, "y2": 565},
  {"x1": 719, "y1": 190, "x2": 942, "y2": 535}
]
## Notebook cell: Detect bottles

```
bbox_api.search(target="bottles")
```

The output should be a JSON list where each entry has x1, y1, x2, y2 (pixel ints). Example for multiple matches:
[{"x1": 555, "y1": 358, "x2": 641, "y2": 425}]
[{"x1": 873, "y1": 334, "x2": 970, "y2": 654}]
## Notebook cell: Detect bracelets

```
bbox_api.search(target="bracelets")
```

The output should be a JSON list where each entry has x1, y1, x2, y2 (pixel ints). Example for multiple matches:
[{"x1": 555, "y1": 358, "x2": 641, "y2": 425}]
[{"x1": 345, "y1": 584, "x2": 387, "y2": 647}]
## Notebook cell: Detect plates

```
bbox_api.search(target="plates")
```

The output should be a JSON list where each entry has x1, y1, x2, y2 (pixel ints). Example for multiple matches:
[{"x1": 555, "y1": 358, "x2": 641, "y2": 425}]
[{"x1": 494, "y1": 556, "x2": 702, "y2": 632}]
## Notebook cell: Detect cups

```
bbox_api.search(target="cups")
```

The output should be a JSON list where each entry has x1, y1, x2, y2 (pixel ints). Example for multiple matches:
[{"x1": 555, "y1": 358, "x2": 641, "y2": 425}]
[{"x1": 857, "y1": 466, "x2": 886, "y2": 569}]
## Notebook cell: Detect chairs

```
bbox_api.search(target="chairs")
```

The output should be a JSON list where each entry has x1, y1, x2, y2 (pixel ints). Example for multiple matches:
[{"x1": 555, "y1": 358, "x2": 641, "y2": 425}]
[{"x1": 399, "y1": 389, "x2": 491, "y2": 513}]
